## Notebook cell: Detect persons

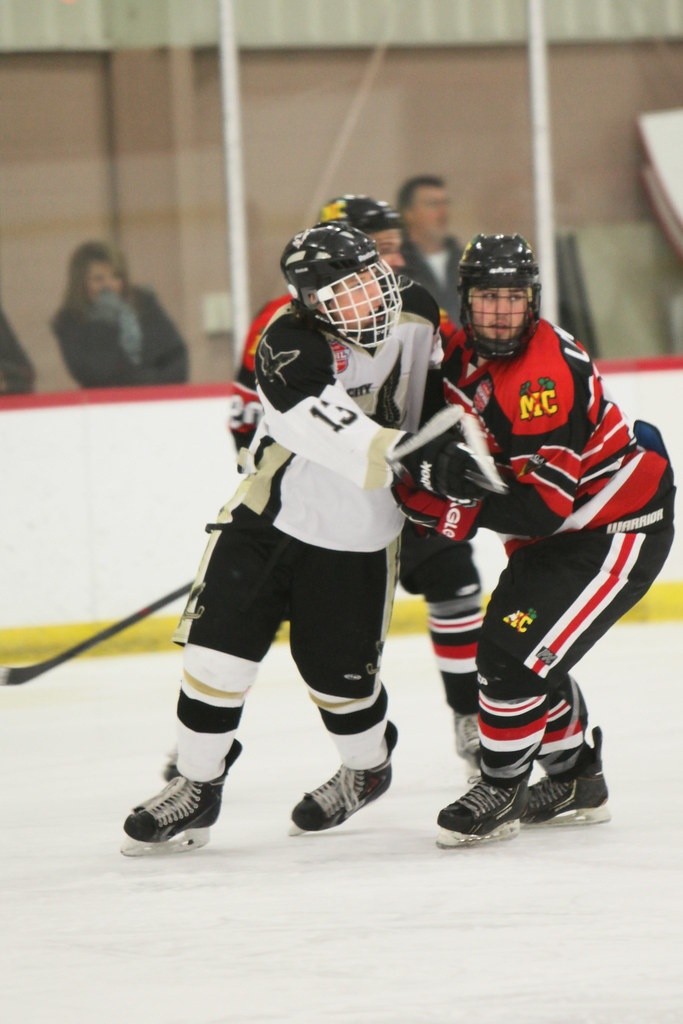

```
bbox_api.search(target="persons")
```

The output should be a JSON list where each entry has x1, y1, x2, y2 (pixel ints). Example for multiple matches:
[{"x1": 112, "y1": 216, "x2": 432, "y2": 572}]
[
  {"x1": 393, "y1": 234, "x2": 678, "y2": 849},
  {"x1": 50, "y1": 239, "x2": 199, "y2": 392},
  {"x1": 394, "y1": 172, "x2": 471, "y2": 331},
  {"x1": 0, "y1": 311, "x2": 39, "y2": 392},
  {"x1": 117, "y1": 221, "x2": 512, "y2": 855},
  {"x1": 228, "y1": 194, "x2": 504, "y2": 784}
]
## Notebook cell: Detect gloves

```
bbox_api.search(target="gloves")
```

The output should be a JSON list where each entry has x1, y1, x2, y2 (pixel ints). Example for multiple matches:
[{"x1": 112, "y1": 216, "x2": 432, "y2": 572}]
[
  {"x1": 394, "y1": 430, "x2": 493, "y2": 503},
  {"x1": 390, "y1": 473, "x2": 484, "y2": 541}
]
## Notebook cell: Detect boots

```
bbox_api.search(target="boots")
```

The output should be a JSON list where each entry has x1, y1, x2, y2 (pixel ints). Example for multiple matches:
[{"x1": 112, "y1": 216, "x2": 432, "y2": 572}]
[
  {"x1": 454, "y1": 713, "x2": 492, "y2": 786},
  {"x1": 289, "y1": 720, "x2": 398, "y2": 836},
  {"x1": 119, "y1": 737, "x2": 242, "y2": 856},
  {"x1": 436, "y1": 742, "x2": 542, "y2": 849},
  {"x1": 520, "y1": 726, "x2": 609, "y2": 828}
]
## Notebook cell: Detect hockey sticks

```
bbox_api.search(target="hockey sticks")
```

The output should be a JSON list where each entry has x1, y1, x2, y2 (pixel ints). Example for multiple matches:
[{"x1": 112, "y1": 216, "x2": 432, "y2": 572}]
[{"x1": 0, "y1": 577, "x2": 198, "y2": 687}]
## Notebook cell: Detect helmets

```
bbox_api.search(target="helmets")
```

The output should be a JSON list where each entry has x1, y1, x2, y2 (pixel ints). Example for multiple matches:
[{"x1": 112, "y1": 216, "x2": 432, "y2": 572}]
[
  {"x1": 319, "y1": 194, "x2": 404, "y2": 238},
  {"x1": 280, "y1": 222, "x2": 379, "y2": 311},
  {"x1": 460, "y1": 233, "x2": 539, "y2": 287}
]
